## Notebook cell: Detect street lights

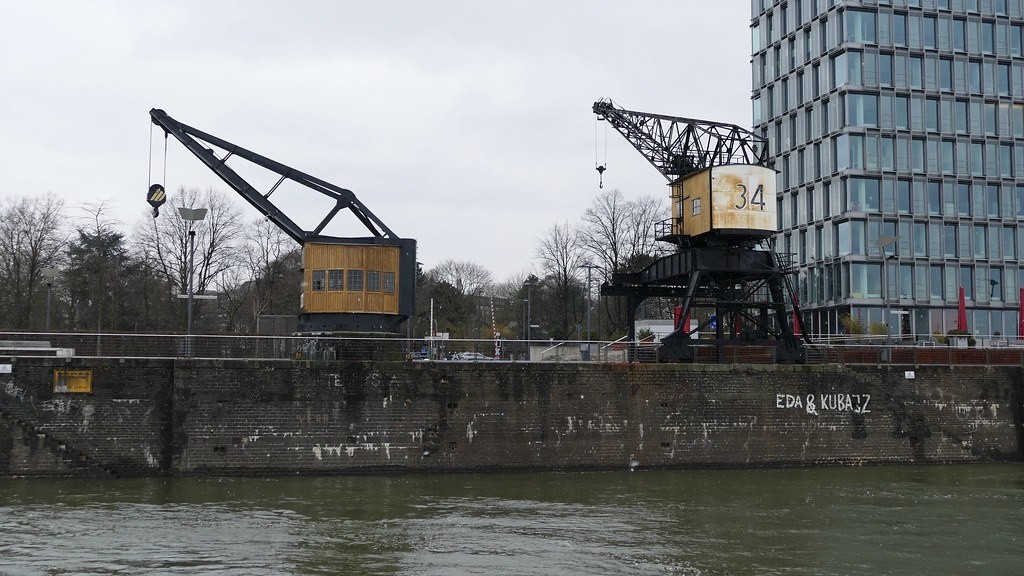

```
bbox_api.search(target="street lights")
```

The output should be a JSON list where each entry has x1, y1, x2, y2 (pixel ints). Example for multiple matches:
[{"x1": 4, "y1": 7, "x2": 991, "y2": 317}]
[
  {"x1": 525, "y1": 282, "x2": 539, "y2": 360},
  {"x1": 578, "y1": 264, "x2": 601, "y2": 361},
  {"x1": 187, "y1": 231, "x2": 196, "y2": 356},
  {"x1": 518, "y1": 298, "x2": 528, "y2": 360},
  {"x1": 47, "y1": 282, "x2": 52, "y2": 333}
]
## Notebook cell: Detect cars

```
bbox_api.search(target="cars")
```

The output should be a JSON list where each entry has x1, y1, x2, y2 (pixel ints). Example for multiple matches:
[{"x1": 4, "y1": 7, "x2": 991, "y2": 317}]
[{"x1": 459, "y1": 351, "x2": 494, "y2": 361}]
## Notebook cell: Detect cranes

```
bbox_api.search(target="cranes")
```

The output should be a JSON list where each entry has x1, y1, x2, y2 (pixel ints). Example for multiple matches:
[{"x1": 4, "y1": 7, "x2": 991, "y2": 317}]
[
  {"x1": 147, "y1": 107, "x2": 417, "y2": 336},
  {"x1": 592, "y1": 97, "x2": 821, "y2": 366}
]
